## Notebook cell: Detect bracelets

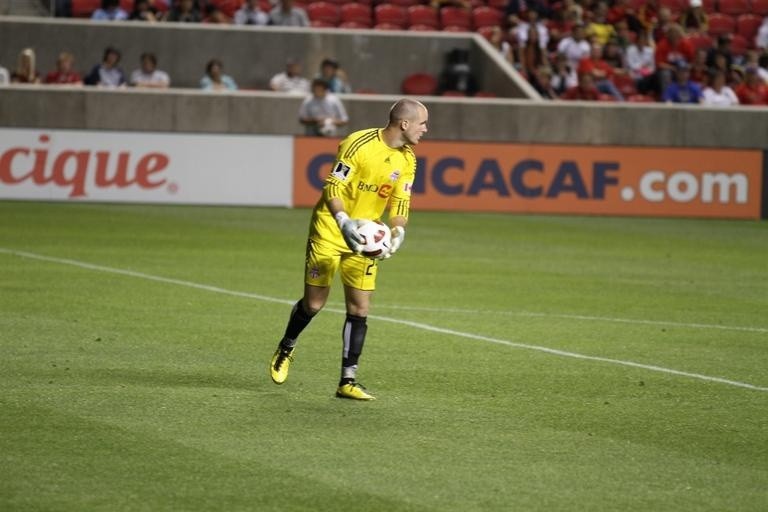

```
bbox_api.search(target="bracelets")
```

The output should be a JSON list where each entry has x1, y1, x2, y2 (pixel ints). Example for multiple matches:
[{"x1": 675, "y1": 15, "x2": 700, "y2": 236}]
[
  {"x1": 392, "y1": 225, "x2": 405, "y2": 233},
  {"x1": 334, "y1": 210, "x2": 351, "y2": 225}
]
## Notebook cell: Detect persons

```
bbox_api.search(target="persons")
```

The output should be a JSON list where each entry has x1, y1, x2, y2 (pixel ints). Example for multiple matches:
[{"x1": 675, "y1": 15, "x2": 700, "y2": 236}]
[
  {"x1": 269, "y1": 97, "x2": 430, "y2": 402},
  {"x1": 269, "y1": 56, "x2": 312, "y2": 92},
  {"x1": 43, "y1": 51, "x2": 85, "y2": 85},
  {"x1": 128, "y1": 51, "x2": 171, "y2": 89},
  {"x1": 198, "y1": 58, "x2": 238, "y2": 89},
  {"x1": 10, "y1": 46, "x2": 42, "y2": 84},
  {"x1": 90, "y1": 0, "x2": 312, "y2": 27},
  {"x1": 81, "y1": 45, "x2": 124, "y2": 86},
  {"x1": 0, "y1": 66, "x2": 11, "y2": 84},
  {"x1": 313, "y1": 57, "x2": 352, "y2": 93},
  {"x1": 298, "y1": 77, "x2": 350, "y2": 135}
]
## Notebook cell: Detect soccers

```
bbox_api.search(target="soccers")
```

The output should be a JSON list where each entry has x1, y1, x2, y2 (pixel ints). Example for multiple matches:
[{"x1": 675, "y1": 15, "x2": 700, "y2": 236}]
[{"x1": 355, "y1": 219, "x2": 393, "y2": 257}]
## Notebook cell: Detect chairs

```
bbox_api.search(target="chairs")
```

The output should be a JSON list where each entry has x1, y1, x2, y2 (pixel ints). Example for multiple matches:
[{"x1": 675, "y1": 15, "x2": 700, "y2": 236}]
[
  {"x1": 401, "y1": 73, "x2": 439, "y2": 97},
  {"x1": 630, "y1": 0, "x2": 768, "y2": 52},
  {"x1": 304, "y1": 0, "x2": 503, "y2": 41}
]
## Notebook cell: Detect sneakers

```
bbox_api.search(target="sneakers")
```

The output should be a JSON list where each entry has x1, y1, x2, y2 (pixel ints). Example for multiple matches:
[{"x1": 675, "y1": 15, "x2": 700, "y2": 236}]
[
  {"x1": 336, "y1": 383, "x2": 376, "y2": 401},
  {"x1": 269, "y1": 338, "x2": 295, "y2": 385}
]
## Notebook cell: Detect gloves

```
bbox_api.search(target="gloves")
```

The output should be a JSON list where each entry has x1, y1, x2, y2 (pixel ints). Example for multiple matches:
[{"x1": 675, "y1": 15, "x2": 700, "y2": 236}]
[
  {"x1": 334, "y1": 211, "x2": 365, "y2": 255},
  {"x1": 383, "y1": 224, "x2": 405, "y2": 258}
]
